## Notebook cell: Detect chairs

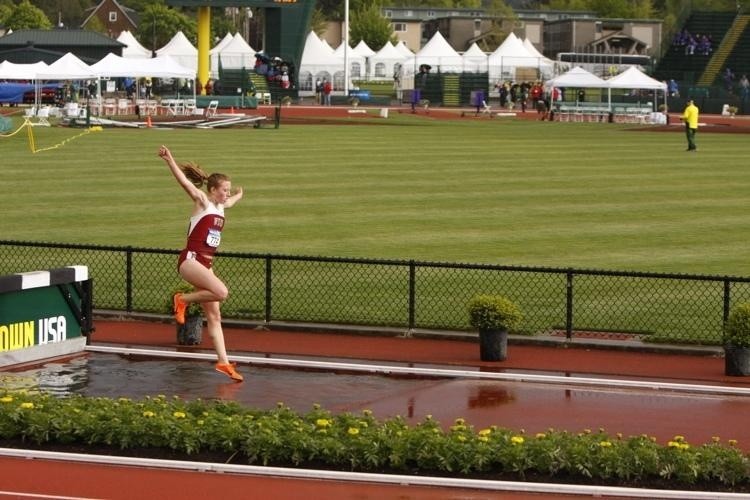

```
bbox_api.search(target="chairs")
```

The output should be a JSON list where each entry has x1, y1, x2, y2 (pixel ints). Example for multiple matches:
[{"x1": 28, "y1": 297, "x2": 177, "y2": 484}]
[
  {"x1": 23, "y1": 98, "x2": 218, "y2": 127},
  {"x1": 548, "y1": 106, "x2": 667, "y2": 123},
  {"x1": 255, "y1": 92, "x2": 271, "y2": 105},
  {"x1": 482, "y1": 100, "x2": 491, "y2": 114}
]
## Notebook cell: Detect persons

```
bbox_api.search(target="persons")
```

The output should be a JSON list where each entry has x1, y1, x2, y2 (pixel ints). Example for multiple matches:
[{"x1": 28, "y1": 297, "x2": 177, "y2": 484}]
[
  {"x1": 60, "y1": 77, "x2": 192, "y2": 108},
  {"x1": 319, "y1": 79, "x2": 328, "y2": 104},
  {"x1": 668, "y1": 95, "x2": 700, "y2": 151},
  {"x1": 669, "y1": 27, "x2": 719, "y2": 56},
  {"x1": 721, "y1": 68, "x2": 750, "y2": 116},
  {"x1": 157, "y1": 143, "x2": 244, "y2": 383},
  {"x1": 324, "y1": 82, "x2": 332, "y2": 107},
  {"x1": 254, "y1": 53, "x2": 294, "y2": 89},
  {"x1": 577, "y1": 87, "x2": 586, "y2": 107},
  {"x1": 628, "y1": 79, "x2": 681, "y2": 98},
  {"x1": 491, "y1": 79, "x2": 566, "y2": 121}
]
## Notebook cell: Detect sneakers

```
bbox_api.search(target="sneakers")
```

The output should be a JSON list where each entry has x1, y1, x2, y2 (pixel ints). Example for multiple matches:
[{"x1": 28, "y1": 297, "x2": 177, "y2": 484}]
[
  {"x1": 174, "y1": 293, "x2": 189, "y2": 324},
  {"x1": 215, "y1": 362, "x2": 244, "y2": 381}
]
